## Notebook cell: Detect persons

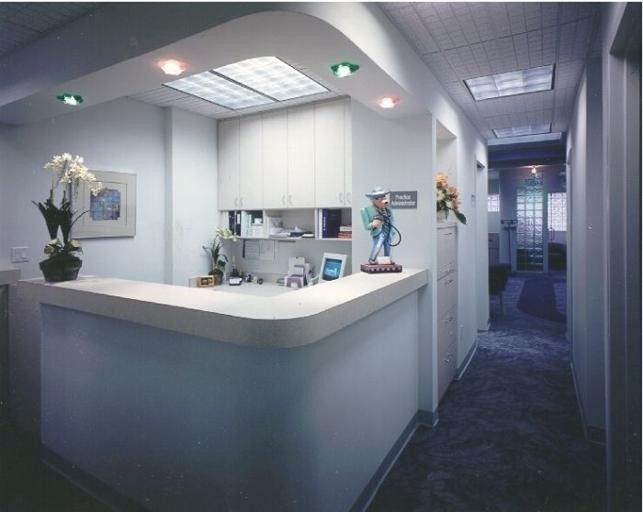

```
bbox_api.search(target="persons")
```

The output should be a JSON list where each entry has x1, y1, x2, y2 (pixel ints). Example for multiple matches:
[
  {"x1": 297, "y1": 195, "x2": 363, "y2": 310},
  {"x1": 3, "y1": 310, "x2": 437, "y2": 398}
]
[{"x1": 359, "y1": 186, "x2": 402, "y2": 273}]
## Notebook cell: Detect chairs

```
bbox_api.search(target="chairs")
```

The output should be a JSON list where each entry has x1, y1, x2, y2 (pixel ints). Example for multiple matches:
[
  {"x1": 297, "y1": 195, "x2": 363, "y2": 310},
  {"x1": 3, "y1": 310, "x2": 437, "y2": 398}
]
[{"x1": 489, "y1": 262, "x2": 511, "y2": 313}]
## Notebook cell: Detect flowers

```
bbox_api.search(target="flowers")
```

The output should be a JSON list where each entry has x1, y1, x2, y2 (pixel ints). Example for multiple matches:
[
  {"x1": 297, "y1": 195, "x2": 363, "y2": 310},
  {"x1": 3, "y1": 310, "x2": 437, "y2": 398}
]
[
  {"x1": 31, "y1": 152, "x2": 103, "y2": 258},
  {"x1": 202, "y1": 226, "x2": 234, "y2": 271},
  {"x1": 436, "y1": 173, "x2": 468, "y2": 225}
]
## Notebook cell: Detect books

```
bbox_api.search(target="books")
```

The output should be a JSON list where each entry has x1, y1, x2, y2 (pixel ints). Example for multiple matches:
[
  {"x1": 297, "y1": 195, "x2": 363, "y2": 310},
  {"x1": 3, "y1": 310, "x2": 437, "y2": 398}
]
[{"x1": 227, "y1": 208, "x2": 351, "y2": 239}]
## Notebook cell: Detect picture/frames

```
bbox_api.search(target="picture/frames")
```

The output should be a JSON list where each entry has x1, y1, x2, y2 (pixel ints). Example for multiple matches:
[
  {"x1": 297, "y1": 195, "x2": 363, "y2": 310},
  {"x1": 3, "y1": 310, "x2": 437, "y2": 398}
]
[{"x1": 69, "y1": 169, "x2": 137, "y2": 240}]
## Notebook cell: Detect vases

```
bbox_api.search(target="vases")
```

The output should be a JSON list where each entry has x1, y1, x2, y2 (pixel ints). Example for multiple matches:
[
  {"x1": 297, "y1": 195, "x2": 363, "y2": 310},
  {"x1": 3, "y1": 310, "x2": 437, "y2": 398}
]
[
  {"x1": 436, "y1": 207, "x2": 450, "y2": 224},
  {"x1": 208, "y1": 269, "x2": 223, "y2": 284},
  {"x1": 37, "y1": 252, "x2": 83, "y2": 282}
]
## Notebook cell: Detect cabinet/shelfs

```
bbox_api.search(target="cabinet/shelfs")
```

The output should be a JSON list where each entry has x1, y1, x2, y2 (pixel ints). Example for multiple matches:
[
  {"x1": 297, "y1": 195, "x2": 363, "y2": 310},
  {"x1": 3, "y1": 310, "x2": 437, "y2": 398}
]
[
  {"x1": 314, "y1": 97, "x2": 353, "y2": 208},
  {"x1": 223, "y1": 208, "x2": 352, "y2": 242},
  {"x1": 437, "y1": 220, "x2": 459, "y2": 405},
  {"x1": 217, "y1": 112, "x2": 264, "y2": 211},
  {"x1": 262, "y1": 102, "x2": 314, "y2": 209}
]
